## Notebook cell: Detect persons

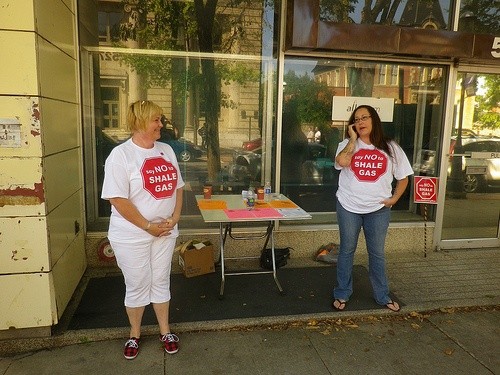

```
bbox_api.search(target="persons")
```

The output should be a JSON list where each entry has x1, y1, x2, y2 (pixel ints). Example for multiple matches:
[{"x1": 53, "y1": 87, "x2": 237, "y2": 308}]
[
  {"x1": 280, "y1": 112, "x2": 308, "y2": 207},
  {"x1": 157, "y1": 115, "x2": 179, "y2": 144},
  {"x1": 332, "y1": 105, "x2": 414, "y2": 311},
  {"x1": 200, "y1": 123, "x2": 208, "y2": 147},
  {"x1": 101, "y1": 100, "x2": 184, "y2": 359}
]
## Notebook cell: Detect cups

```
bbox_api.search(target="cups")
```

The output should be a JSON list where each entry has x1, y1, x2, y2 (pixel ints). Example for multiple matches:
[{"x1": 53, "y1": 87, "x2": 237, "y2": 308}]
[
  {"x1": 203, "y1": 185, "x2": 212, "y2": 199},
  {"x1": 258, "y1": 190, "x2": 264, "y2": 201},
  {"x1": 242, "y1": 191, "x2": 248, "y2": 202}
]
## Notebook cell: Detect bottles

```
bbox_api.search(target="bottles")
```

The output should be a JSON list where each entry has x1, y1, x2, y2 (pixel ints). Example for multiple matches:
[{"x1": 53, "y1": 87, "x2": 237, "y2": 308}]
[
  {"x1": 264, "y1": 182, "x2": 271, "y2": 202},
  {"x1": 247, "y1": 187, "x2": 255, "y2": 209}
]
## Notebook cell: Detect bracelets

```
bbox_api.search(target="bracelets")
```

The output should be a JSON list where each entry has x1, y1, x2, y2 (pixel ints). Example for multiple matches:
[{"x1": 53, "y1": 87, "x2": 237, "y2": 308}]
[{"x1": 146, "y1": 222, "x2": 151, "y2": 230}]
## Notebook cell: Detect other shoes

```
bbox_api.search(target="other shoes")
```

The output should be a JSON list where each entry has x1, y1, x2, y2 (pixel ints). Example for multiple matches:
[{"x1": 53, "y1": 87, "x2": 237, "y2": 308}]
[
  {"x1": 160, "y1": 332, "x2": 179, "y2": 354},
  {"x1": 124, "y1": 338, "x2": 140, "y2": 360}
]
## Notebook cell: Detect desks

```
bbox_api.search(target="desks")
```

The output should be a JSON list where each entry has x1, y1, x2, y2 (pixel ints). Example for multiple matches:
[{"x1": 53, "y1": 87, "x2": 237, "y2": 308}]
[{"x1": 194, "y1": 193, "x2": 312, "y2": 301}]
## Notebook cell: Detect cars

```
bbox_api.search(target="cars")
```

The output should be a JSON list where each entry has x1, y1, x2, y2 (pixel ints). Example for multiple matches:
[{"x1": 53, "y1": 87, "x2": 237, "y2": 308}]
[
  {"x1": 304, "y1": 140, "x2": 341, "y2": 193},
  {"x1": 234, "y1": 136, "x2": 265, "y2": 181},
  {"x1": 101, "y1": 132, "x2": 122, "y2": 161},
  {"x1": 446, "y1": 135, "x2": 500, "y2": 194},
  {"x1": 155, "y1": 117, "x2": 202, "y2": 163}
]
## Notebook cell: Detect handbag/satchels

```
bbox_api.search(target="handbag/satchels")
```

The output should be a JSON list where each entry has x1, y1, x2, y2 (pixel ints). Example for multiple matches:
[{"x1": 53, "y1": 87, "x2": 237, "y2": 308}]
[
  {"x1": 198, "y1": 128, "x2": 203, "y2": 137},
  {"x1": 260, "y1": 247, "x2": 295, "y2": 271}
]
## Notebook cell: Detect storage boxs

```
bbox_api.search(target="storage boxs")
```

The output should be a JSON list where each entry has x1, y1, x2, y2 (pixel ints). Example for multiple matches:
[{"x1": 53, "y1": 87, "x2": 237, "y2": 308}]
[{"x1": 179, "y1": 239, "x2": 216, "y2": 279}]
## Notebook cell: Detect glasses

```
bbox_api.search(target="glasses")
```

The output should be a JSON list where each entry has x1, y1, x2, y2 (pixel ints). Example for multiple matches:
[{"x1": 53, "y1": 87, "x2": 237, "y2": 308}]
[{"x1": 352, "y1": 116, "x2": 371, "y2": 123}]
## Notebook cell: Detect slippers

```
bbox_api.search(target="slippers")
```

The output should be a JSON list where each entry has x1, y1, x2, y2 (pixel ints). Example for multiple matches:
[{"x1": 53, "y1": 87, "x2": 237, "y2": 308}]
[
  {"x1": 385, "y1": 300, "x2": 401, "y2": 312},
  {"x1": 332, "y1": 298, "x2": 348, "y2": 310}
]
